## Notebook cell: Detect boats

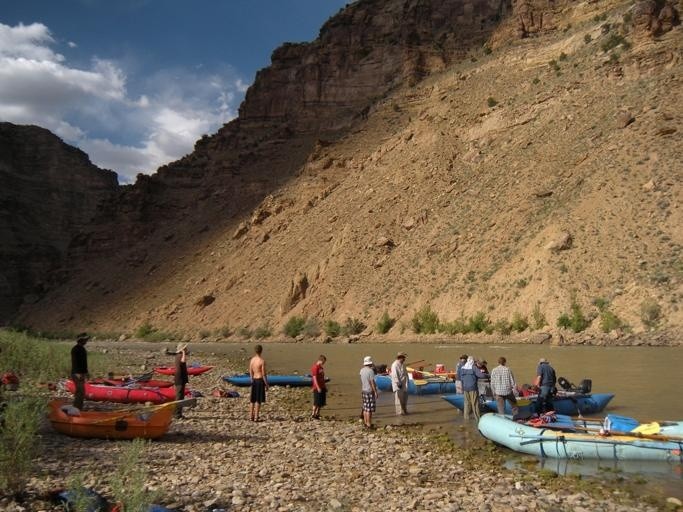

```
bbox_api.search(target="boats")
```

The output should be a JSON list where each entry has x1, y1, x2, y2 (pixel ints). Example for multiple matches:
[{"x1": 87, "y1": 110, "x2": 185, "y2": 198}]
[
  {"x1": 476, "y1": 415, "x2": 683, "y2": 462},
  {"x1": 149, "y1": 361, "x2": 215, "y2": 376},
  {"x1": 440, "y1": 375, "x2": 616, "y2": 413},
  {"x1": 45, "y1": 395, "x2": 198, "y2": 442},
  {"x1": 63, "y1": 373, "x2": 198, "y2": 405},
  {"x1": 373, "y1": 373, "x2": 454, "y2": 394},
  {"x1": 221, "y1": 373, "x2": 331, "y2": 387}
]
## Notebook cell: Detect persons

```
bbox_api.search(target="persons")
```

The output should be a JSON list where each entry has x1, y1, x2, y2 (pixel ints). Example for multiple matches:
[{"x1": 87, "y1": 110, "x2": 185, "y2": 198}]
[
  {"x1": 389, "y1": 351, "x2": 409, "y2": 416},
  {"x1": 491, "y1": 356, "x2": 516, "y2": 416},
  {"x1": 68, "y1": 333, "x2": 91, "y2": 406},
  {"x1": 533, "y1": 357, "x2": 558, "y2": 414},
  {"x1": 172, "y1": 342, "x2": 191, "y2": 420},
  {"x1": 452, "y1": 353, "x2": 489, "y2": 420},
  {"x1": 247, "y1": 345, "x2": 267, "y2": 422},
  {"x1": 310, "y1": 355, "x2": 331, "y2": 421},
  {"x1": 356, "y1": 356, "x2": 379, "y2": 431}
]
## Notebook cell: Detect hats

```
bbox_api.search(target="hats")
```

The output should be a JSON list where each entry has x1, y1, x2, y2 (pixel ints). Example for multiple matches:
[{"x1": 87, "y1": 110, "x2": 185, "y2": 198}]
[
  {"x1": 460, "y1": 355, "x2": 467, "y2": 360},
  {"x1": 77, "y1": 333, "x2": 90, "y2": 343},
  {"x1": 538, "y1": 358, "x2": 549, "y2": 364},
  {"x1": 363, "y1": 356, "x2": 373, "y2": 365},
  {"x1": 176, "y1": 342, "x2": 188, "y2": 353},
  {"x1": 398, "y1": 351, "x2": 408, "y2": 358}
]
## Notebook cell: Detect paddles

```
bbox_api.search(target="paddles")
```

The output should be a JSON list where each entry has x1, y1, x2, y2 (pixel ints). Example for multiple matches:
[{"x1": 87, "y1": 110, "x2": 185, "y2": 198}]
[
  {"x1": 511, "y1": 433, "x2": 681, "y2": 451},
  {"x1": 422, "y1": 372, "x2": 454, "y2": 377},
  {"x1": 414, "y1": 380, "x2": 453, "y2": 385},
  {"x1": 406, "y1": 366, "x2": 430, "y2": 373},
  {"x1": 91, "y1": 398, "x2": 197, "y2": 426}
]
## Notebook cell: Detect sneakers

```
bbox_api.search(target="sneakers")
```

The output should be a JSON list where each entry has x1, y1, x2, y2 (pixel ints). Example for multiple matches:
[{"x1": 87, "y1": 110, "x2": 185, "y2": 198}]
[{"x1": 359, "y1": 414, "x2": 377, "y2": 430}]
